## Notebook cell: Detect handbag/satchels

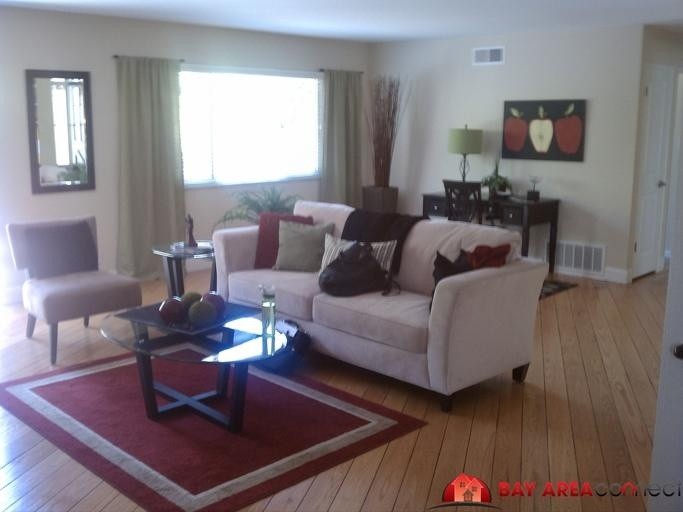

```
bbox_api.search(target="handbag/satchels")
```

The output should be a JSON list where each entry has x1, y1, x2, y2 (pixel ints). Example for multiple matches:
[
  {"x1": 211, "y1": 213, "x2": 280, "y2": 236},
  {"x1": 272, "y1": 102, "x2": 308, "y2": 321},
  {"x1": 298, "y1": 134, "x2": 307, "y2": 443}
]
[{"x1": 318, "y1": 242, "x2": 402, "y2": 297}]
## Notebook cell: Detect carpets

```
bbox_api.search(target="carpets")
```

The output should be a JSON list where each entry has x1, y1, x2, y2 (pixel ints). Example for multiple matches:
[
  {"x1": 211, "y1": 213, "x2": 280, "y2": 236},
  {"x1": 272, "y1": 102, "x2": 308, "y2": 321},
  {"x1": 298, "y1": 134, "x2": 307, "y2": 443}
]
[{"x1": 0, "y1": 332, "x2": 427, "y2": 512}]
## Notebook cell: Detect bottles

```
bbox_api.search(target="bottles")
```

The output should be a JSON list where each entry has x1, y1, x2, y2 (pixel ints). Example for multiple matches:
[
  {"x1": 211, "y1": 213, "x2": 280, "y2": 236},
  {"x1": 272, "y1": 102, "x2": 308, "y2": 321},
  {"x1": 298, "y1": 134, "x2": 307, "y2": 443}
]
[{"x1": 262, "y1": 293, "x2": 276, "y2": 336}]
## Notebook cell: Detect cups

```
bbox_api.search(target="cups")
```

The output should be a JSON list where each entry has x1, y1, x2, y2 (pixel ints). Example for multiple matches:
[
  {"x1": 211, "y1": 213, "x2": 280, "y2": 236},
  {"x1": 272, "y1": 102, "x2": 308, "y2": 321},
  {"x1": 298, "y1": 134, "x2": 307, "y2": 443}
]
[{"x1": 115, "y1": 294, "x2": 263, "y2": 336}]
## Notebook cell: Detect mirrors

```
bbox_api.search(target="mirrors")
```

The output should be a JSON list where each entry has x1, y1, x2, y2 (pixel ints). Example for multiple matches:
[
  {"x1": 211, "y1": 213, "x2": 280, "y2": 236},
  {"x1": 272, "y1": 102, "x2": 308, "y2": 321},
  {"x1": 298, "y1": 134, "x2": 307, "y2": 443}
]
[{"x1": 24, "y1": 69, "x2": 96, "y2": 196}]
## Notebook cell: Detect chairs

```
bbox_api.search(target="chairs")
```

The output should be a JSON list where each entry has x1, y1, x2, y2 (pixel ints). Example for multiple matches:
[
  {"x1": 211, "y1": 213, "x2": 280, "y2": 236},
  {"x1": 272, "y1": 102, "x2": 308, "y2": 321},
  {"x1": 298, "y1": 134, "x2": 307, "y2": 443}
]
[
  {"x1": 151, "y1": 239, "x2": 217, "y2": 297},
  {"x1": 6, "y1": 215, "x2": 142, "y2": 363},
  {"x1": 442, "y1": 179, "x2": 481, "y2": 224}
]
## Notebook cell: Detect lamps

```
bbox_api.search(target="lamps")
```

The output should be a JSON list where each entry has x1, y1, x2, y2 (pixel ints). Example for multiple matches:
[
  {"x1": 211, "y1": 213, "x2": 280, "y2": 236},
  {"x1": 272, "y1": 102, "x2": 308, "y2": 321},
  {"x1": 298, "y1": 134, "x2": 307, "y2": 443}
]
[{"x1": 448, "y1": 124, "x2": 482, "y2": 180}]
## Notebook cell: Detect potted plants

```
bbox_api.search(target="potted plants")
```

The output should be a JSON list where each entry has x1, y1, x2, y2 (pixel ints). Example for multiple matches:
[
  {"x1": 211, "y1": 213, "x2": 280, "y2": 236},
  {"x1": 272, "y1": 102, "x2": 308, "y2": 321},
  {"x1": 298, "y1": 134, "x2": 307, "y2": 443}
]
[
  {"x1": 526, "y1": 176, "x2": 541, "y2": 200},
  {"x1": 216, "y1": 187, "x2": 308, "y2": 229},
  {"x1": 482, "y1": 161, "x2": 511, "y2": 200},
  {"x1": 361, "y1": 73, "x2": 412, "y2": 213}
]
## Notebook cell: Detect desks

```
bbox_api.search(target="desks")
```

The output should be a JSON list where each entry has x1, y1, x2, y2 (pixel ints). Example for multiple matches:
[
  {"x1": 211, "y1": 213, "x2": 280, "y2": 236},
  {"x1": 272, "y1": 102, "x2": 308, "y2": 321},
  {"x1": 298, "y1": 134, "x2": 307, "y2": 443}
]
[{"x1": 421, "y1": 191, "x2": 560, "y2": 277}]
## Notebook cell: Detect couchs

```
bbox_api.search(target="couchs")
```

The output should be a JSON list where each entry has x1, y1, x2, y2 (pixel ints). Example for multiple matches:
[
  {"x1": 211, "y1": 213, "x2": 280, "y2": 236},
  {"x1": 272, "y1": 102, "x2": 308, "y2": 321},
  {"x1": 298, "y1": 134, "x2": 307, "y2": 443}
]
[{"x1": 211, "y1": 198, "x2": 550, "y2": 412}]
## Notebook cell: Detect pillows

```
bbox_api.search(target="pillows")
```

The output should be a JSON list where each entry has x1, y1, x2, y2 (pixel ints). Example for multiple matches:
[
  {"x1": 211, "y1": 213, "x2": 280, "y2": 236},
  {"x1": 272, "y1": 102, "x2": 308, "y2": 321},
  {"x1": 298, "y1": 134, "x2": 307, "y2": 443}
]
[
  {"x1": 428, "y1": 248, "x2": 469, "y2": 314},
  {"x1": 318, "y1": 232, "x2": 398, "y2": 276},
  {"x1": 271, "y1": 219, "x2": 335, "y2": 272},
  {"x1": 467, "y1": 243, "x2": 511, "y2": 269},
  {"x1": 253, "y1": 211, "x2": 314, "y2": 269}
]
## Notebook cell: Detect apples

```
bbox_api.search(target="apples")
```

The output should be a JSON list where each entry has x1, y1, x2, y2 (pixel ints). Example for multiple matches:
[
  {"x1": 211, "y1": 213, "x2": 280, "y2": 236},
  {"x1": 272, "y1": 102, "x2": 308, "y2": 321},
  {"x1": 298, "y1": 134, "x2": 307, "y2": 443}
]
[
  {"x1": 504, "y1": 117, "x2": 528, "y2": 152},
  {"x1": 554, "y1": 111, "x2": 581, "y2": 154},
  {"x1": 529, "y1": 118, "x2": 554, "y2": 154}
]
